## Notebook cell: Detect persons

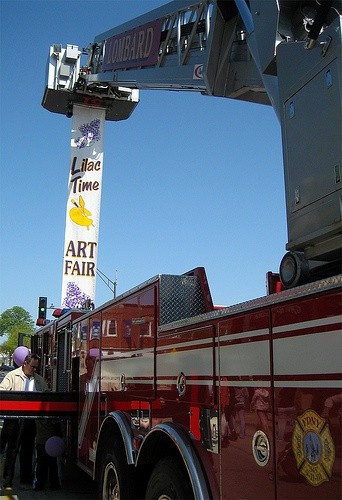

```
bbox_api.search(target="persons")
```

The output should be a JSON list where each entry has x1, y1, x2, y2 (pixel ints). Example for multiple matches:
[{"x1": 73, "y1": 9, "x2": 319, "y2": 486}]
[
  {"x1": 177, "y1": 369, "x2": 342, "y2": 480},
  {"x1": 0, "y1": 353, "x2": 51, "y2": 488},
  {"x1": 31, "y1": 390, "x2": 65, "y2": 490}
]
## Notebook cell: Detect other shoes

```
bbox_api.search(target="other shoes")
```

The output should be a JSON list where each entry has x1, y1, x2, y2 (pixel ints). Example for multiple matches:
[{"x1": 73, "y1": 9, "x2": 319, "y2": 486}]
[
  {"x1": 18, "y1": 484, "x2": 33, "y2": 490},
  {"x1": 2, "y1": 480, "x2": 13, "y2": 490}
]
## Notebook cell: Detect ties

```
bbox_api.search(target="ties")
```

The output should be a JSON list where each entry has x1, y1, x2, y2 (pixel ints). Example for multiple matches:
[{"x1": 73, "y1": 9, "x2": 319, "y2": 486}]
[{"x1": 25, "y1": 376, "x2": 30, "y2": 392}]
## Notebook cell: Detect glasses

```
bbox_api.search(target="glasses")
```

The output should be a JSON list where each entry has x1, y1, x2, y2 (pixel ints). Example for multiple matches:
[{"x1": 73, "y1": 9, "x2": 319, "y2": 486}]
[{"x1": 27, "y1": 362, "x2": 39, "y2": 370}]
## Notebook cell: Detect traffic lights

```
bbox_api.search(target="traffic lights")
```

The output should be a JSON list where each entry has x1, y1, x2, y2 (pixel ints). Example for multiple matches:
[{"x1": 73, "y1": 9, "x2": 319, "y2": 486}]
[{"x1": 37, "y1": 297, "x2": 47, "y2": 325}]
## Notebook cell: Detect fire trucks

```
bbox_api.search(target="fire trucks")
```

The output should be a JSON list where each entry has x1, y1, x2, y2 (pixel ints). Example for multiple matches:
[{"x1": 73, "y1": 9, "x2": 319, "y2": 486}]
[{"x1": 18, "y1": 0, "x2": 342, "y2": 500}]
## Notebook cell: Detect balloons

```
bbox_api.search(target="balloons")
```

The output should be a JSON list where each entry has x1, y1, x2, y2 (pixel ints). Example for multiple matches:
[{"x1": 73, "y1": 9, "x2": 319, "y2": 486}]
[
  {"x1": 44, "y1": 436, "x2": 66, "y2": 457},
  {"x1": 12, "y1": 345, "x2": 31, "y2": 366}
]
